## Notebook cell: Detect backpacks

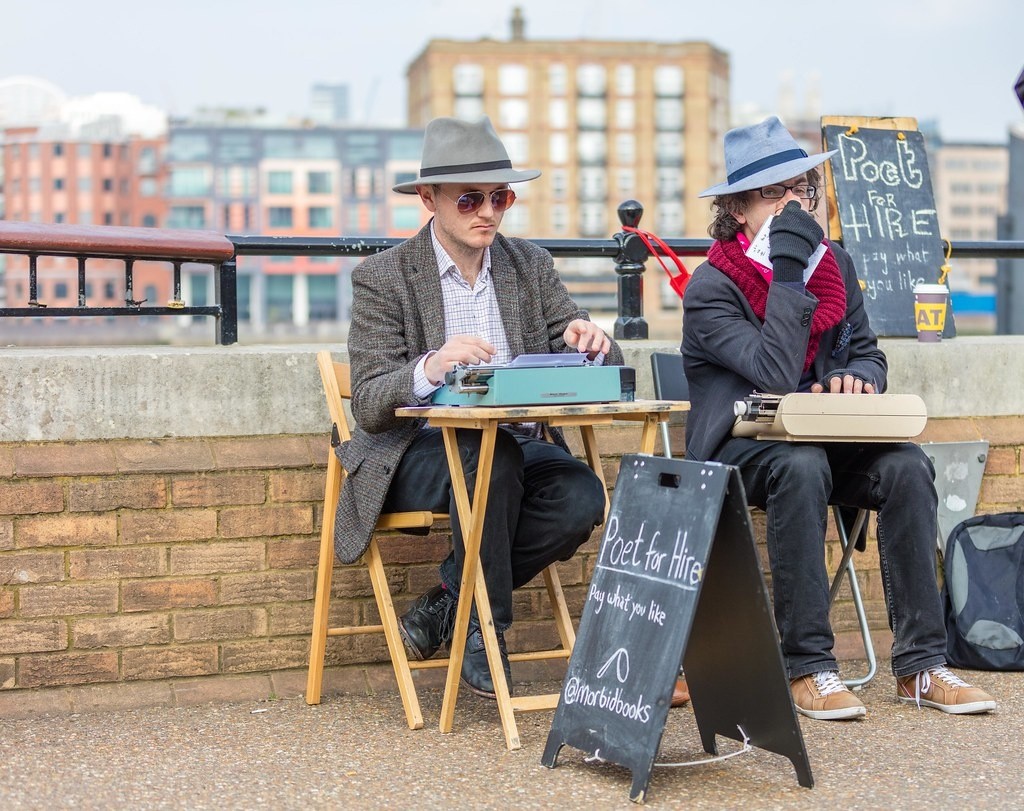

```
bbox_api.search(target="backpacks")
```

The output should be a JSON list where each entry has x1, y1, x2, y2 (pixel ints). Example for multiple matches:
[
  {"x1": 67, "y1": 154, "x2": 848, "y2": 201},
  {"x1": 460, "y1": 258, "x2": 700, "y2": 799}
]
[{"x1": 939, "y1": 512, "x2": 1024, "y2": 672}]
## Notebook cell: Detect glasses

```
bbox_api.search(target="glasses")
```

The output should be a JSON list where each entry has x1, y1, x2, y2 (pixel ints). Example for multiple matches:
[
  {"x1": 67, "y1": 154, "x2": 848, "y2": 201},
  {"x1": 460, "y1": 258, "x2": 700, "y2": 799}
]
[
  {"x1": 748, "y1": 184, "x2": 816, "y2": 199},
  {"x1": 434, "y1": 184, "x2": 516, "y2": 216}
]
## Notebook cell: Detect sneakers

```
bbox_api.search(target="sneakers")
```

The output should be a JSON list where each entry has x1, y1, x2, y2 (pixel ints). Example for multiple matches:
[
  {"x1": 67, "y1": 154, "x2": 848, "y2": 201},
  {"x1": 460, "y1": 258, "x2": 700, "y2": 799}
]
[
  {"x1": 788, "y1": 670, "x2": 867, "y2": 719},
  {"x1": 896, "y1": 664, "x2": 997, "y2": 714}
]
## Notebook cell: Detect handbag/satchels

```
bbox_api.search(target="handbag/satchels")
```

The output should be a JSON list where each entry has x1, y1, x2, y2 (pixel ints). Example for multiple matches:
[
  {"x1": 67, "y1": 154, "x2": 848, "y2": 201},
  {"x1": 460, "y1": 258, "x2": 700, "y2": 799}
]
[{"x1": 621, "y1": 223, "x2": 692, "y2": 300}]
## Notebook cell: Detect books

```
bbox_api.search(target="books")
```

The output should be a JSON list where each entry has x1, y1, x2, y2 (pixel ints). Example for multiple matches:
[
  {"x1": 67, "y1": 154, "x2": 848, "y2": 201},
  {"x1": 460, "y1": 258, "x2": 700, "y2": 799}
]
[{"x1": 745, "y1": 214, "x2": 829, "y2": 287}]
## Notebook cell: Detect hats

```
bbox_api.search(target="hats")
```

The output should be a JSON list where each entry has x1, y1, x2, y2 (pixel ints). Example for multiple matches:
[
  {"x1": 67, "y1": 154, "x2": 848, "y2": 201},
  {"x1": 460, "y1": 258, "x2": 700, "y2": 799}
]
[
  {"x1": 392, "y1": 115, "x2": 543, "y2": 195},
  {"x1": 696, "y1": 115, "x2": 839, "y2": 198}
]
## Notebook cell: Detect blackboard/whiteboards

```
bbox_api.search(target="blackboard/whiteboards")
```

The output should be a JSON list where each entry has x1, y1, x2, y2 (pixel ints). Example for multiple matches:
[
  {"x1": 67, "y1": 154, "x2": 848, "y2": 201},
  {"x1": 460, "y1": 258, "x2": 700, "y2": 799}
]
[
  {"x1": 820, "y1": 116, "x2": 957, "y2": 339},
  {"x1": 551, "y1": 452, "x2": 730, "y2": 771}
]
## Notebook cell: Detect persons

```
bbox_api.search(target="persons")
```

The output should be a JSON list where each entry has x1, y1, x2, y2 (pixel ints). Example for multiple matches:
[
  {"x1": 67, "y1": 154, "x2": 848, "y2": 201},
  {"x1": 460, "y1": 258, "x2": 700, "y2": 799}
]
[
  {"x1": 334, "y1": 116, "x2": 626, "y2": 699},
  {"x1": 679, "y1": 116, "x2": 995, "y2": 720}
]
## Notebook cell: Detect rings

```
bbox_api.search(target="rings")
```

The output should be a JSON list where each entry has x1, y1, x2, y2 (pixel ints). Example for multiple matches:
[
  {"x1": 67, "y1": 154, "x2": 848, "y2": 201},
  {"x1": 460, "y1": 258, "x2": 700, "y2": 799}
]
[{"x1": 775, "y1": 207, "x2": 784, "y2": 214}]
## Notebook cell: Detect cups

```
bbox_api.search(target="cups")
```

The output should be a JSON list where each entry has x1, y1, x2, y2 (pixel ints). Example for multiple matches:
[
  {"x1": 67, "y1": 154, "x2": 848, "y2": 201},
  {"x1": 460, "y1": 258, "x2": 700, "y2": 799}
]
[{"x1": 913, "y1": 284, "x2": 949, "y2": 342}]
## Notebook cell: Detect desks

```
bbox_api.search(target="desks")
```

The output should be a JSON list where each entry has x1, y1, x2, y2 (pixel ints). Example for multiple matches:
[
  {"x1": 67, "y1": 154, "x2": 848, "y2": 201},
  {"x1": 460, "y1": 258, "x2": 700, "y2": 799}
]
[{"x1": 395, "y1": 400, "x2": 692, "y2": 751}]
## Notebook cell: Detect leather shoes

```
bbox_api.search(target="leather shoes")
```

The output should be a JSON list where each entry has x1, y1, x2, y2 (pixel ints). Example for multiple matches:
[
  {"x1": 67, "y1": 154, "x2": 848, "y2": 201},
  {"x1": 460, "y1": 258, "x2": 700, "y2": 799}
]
[
  {"x1": 447, "y1": 617, "x2": 514, "y2": 698},
  {"x1": 397, "y1": 583, "x2": 458, "y2": 661}
]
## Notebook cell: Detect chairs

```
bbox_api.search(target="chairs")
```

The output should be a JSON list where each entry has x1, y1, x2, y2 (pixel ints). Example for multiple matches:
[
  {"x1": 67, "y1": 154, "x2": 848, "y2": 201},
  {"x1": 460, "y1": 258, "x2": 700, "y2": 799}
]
[
  {"x1": 305, "y1": 350, "x2": 576, "y2": 730},
  {"x1": 651, "y1": 352, "x2": 877, "y2": 688}
]
